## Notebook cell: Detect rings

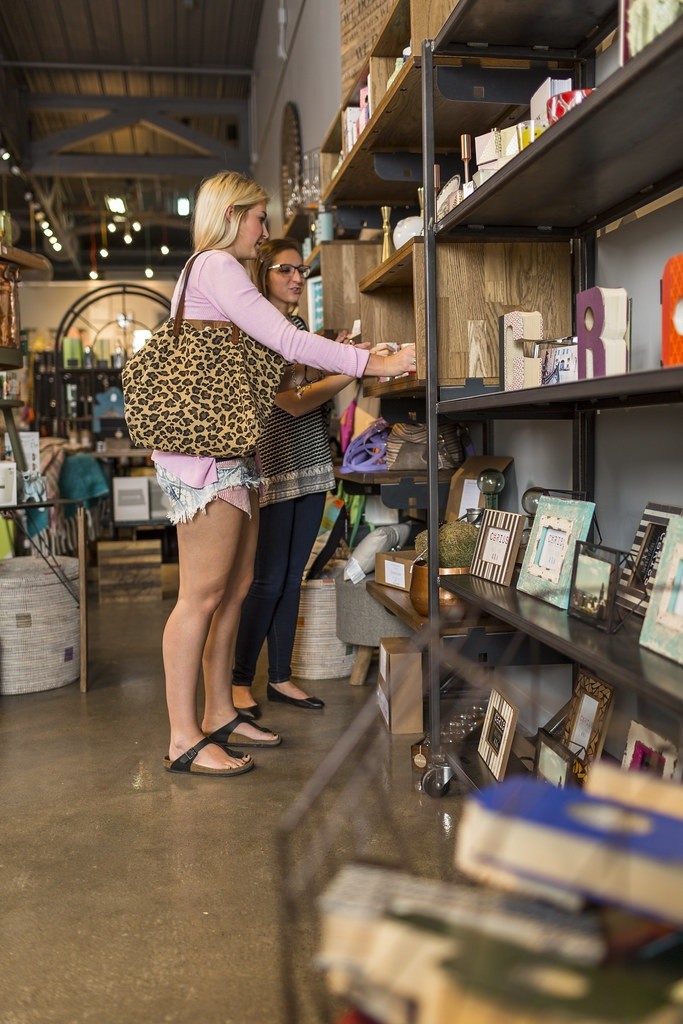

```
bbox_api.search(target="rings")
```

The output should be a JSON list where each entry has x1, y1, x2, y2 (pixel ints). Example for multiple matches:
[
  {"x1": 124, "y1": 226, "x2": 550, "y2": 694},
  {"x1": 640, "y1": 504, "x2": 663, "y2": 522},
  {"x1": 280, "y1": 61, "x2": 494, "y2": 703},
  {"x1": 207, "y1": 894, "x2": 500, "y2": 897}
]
[{"x1": 414, "y1": 360, "x2": 416, "y2": 365}]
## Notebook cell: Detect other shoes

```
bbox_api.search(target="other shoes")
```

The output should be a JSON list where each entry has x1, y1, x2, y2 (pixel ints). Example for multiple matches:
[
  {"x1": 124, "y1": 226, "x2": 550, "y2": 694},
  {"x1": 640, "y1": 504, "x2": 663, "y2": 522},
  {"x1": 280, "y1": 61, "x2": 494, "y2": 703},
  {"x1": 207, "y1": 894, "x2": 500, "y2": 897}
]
[
  {"x1": 234, "y1": 703, "x2": 261, "y2": 720},
  {"x1": 267, "y1": 682, "x2": 324, "y2": 709}
]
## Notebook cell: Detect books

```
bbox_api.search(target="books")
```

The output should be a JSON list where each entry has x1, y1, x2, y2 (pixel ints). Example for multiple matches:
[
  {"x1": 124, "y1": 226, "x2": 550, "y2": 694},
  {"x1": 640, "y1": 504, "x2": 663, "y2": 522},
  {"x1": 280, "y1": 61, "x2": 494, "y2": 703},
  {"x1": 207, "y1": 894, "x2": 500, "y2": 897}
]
[
  {"x1": 320, "y1": 766, "x2": 683, "y2": 1024},
  {"x1": 530, "y1": 77, "x2": 572, "y2": 119},
  {"x1": 505, "y1": 286, "x2": 629, "y2": 391},
  {"x1": 661, "y1": 252, "x2": 683, "y2": 367}
]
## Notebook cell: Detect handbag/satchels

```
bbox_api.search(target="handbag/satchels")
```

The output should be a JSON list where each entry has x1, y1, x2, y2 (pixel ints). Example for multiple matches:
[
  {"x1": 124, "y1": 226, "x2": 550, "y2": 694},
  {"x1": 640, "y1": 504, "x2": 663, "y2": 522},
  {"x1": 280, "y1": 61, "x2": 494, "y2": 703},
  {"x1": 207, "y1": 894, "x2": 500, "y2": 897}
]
[
  {"x1": 120, "y1": 248, "x2": 293, "y2": 458},
  {"x1": 338, "y1": 411, "x2": 475, "y2": 474}
]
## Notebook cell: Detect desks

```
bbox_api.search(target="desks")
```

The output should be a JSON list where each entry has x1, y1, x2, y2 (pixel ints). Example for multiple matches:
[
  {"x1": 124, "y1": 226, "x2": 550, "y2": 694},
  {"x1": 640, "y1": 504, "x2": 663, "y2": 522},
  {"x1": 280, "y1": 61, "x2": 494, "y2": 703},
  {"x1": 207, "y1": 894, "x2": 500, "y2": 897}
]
[
  {"x1": 334, "y1": 466, "x2": 460, "y2": 533},
  {"x1": 0, "y1": 498, "x2": 90, "y2": 692}
]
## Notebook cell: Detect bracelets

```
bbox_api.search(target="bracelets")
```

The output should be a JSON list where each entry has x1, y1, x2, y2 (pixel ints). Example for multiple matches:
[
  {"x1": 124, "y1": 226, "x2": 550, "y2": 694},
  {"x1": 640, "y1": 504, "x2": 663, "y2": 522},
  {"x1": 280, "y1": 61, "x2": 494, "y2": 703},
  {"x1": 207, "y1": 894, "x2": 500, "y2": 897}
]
[
  {"x1": 305, "y1": 365, "x2": 312, "y2": 384},
  {"x1": 291, "y1": 363, "x2": 305, "y2": 398}
]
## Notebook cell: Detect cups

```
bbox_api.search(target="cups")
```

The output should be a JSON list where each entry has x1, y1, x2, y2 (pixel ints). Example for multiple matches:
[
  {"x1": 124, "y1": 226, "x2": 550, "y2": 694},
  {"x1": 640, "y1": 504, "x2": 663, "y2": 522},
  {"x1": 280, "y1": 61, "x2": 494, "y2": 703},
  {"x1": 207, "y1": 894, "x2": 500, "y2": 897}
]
[
  {"x1": 318, "y1": 214, "x2": 333, "y2": 243},
  {"x1": 515, "y1": 119, "x2": 550, "y2": 152}
]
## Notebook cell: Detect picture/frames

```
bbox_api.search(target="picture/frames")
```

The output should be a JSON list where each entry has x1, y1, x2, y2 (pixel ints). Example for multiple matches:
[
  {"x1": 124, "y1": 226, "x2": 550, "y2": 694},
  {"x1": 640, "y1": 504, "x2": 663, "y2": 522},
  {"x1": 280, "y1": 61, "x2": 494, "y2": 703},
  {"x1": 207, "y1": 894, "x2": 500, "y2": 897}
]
[
  {"x1": 468, "y1": 494, "x2": 683, "y2": 666},
  {"x1": 477, "y1": 669, "x2": 683, "y2": 786}
]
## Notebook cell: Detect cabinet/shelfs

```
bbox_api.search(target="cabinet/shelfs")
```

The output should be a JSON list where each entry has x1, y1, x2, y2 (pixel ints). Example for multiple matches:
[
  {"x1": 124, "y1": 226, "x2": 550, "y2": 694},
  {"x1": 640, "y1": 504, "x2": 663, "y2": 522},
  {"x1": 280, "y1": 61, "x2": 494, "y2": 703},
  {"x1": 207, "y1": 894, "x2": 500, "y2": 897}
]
[
  {"x1": 280, "y1": 0, "x2": 579, "y2": 396},
  {"x1": 425, "y1": 24, "x2": 683, "y2": 806}
]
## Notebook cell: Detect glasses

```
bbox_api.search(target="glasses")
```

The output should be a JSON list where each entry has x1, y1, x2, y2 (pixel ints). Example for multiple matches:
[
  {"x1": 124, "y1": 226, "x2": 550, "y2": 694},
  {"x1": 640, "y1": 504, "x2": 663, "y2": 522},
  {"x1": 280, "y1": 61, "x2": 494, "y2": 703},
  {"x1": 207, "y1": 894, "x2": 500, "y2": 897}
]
[{"x1": 269, "y1": 264, "x2": 311, "y2": 279}]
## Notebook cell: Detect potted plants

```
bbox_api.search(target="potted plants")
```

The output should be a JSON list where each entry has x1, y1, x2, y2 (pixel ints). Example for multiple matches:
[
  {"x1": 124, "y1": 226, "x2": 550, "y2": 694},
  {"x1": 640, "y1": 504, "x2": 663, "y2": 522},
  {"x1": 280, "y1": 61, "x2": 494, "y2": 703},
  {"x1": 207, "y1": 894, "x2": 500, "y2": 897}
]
[
  {"x1": 410, "y1": 529, "x2": 430, "y2": 617},
  {"x1": 438, "y1": 519, "x2": 478, "y2": 625}
]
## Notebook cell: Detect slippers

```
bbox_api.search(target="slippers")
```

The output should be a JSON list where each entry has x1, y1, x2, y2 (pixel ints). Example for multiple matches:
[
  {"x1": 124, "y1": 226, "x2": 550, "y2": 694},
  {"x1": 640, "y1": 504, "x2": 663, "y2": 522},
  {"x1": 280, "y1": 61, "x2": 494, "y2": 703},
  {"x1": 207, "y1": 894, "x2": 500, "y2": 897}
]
[
  {"x1": 200, "y1": 712, "x2": 282, "y2": 748},
  {"x1": 162, "y1": 737, "x2": 254, "y2": 776}
]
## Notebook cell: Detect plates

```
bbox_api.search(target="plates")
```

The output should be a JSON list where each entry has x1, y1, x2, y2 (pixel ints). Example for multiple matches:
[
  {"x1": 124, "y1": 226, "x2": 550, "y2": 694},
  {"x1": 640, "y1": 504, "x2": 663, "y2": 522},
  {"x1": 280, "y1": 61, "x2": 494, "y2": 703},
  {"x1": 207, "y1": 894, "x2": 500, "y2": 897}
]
[{"x1": 437, "y1": 174, "x2": 463, "y2": 211}]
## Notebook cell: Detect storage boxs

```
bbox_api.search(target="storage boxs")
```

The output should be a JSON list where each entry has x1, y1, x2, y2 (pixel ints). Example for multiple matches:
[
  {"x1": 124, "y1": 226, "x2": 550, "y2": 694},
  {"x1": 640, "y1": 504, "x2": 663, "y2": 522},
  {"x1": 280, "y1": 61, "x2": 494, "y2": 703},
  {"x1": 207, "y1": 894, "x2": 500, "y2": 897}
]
[
  {"x1": 374, "y1": 549, "x2": 418, "y2": 592},
  {"x1": 376, "y1": 636, "x2": 425, "y2": 734},
  {"x1": 474, "y1": 124, "x2": 519, "y2": 169},
  {"x1": 112, "y1": 476, "x2": 172, "y2": 520}
]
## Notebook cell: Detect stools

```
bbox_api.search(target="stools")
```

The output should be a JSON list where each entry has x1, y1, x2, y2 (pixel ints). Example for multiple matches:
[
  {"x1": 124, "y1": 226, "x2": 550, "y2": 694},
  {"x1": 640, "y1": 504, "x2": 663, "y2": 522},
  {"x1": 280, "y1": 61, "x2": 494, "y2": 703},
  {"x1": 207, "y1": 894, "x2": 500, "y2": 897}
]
[{"x1": 335, "y1": 570, "x2": 417, "y2": 686}]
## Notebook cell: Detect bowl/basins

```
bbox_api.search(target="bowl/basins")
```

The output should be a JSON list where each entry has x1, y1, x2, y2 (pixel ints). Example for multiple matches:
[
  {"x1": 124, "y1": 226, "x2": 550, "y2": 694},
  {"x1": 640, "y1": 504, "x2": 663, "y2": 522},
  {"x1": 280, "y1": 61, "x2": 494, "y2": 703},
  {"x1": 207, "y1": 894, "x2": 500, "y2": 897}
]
[{"x1": 545, "y1": 87, "x2": 596, "y2": 127}]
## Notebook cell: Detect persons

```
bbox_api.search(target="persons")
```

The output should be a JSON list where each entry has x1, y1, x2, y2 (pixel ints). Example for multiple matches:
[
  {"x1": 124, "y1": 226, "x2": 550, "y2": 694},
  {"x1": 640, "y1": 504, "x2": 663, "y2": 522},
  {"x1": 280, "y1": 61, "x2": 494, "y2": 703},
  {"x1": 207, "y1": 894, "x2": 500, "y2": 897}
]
[
  {"x1": 230, "y1": 238, "x2": 397, "y2": 719},
  {"x1": 151, "y1": 172, "x2": 417, "y2": 774}
]
